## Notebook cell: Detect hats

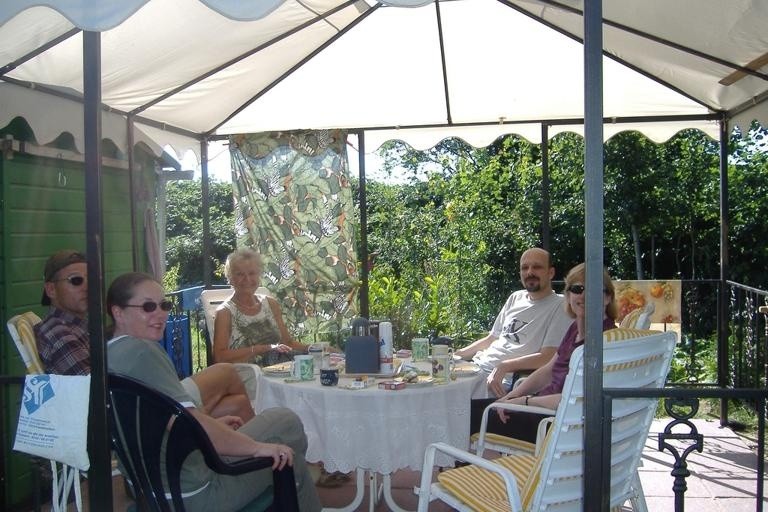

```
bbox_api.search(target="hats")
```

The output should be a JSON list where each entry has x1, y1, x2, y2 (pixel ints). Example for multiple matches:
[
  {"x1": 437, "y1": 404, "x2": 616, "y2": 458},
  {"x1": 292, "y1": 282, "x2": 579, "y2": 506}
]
[{"x1": 40, "y1": 247, "x2": 88, "y2": 307}]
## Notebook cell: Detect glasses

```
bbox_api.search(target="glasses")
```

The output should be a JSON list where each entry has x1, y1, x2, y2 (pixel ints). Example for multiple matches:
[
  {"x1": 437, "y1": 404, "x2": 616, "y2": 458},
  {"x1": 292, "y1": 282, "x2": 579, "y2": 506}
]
[
  {"x1": 122, "y1": 299, "x2": 174, "y2": 316},
  {"x1": 51, "y1": 276, "x2": 94, "y2": 290},
  {"x1": 567, "y1": 283, "x2": 607, "y2": 296}
]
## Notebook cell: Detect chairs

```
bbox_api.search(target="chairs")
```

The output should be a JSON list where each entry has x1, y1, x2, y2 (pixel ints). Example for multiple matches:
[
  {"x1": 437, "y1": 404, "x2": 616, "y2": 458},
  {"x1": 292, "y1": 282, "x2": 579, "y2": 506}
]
[
  {"x1": 418, "y1": 327, "x2": 676, "y2": 512},
  {"x1": 7, "y1": 310, "x2": 123, "y2": 512},
  {"x1": 201, "y1": 287, "x2": 272, "y2": 416},
  {"x1": 471, "y1": 302, "x2": 656, "y2": 457},
  {"x1": 106, "y1": 372, "x2": 300, "y2": 511}
]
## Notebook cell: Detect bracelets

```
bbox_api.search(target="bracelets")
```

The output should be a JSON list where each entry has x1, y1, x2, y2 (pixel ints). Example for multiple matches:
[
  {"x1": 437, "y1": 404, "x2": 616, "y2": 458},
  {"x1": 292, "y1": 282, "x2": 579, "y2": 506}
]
[
  {"x1": 249, "y1": 343, "x2": 258, "y2": 358},
  {"x1": 524, "y1": 392, "x2": 533, "y2": 407}
]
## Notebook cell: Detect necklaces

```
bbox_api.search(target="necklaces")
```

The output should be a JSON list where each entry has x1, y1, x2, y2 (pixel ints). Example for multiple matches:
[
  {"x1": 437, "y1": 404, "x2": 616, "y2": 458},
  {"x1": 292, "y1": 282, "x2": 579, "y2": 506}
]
[{"x1": 230, "y1": 293, "x2": 260, "y2": 314}]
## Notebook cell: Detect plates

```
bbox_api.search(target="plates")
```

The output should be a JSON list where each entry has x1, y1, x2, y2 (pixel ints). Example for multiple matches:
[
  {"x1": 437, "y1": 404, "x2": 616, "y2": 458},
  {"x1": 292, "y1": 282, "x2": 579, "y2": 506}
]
[
  {"x1": 394, "y1": 376, "x2": 433, "y2": 386},
  {"x1": 450, "y1": 363, "x2": 481, "y2": 377},
  {"x1": 428, "y1": 354, "x2": 461, "y2": 363}
]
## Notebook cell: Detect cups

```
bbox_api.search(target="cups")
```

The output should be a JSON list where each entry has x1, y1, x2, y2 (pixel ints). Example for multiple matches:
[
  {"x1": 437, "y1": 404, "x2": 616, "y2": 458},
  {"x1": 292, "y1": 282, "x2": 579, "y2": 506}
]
[
  {"x1": 290, "y1": 355, "x2": 314, "y2": 380},
  {"x1": 432, "y1": 345, "x2": 456, "y2": 378},
  {"x1": 411, "y1": 338, "x2": 429, "y2": 361},
  {"x1": 320, "y1": 368, "x2": 339, "y2": 385}
]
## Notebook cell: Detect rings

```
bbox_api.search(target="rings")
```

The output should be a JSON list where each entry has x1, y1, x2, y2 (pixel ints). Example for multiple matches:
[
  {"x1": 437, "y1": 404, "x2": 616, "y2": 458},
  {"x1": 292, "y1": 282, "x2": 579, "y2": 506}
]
[{"x1": 280, "y1": 451, "x2": 287, "y2": 457}]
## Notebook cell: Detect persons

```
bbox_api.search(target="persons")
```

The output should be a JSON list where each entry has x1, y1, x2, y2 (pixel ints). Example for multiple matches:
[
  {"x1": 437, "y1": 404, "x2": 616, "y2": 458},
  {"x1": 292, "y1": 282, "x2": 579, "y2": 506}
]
[
  {"x1": 448, "y1": 246, "x2": 572, "y2": 435},
  {"x1": 106, "y1": 271, "x2": 326, "y2": 512},
  {"x1": 32, "y1": 249, "x2": 256, "y2": 426},
  {"x1": 471, "y1": 263, "x2": 618, "y2": 445},
  {"x1": 213, "y1": 250, "x2": 341, "y2": 366}
]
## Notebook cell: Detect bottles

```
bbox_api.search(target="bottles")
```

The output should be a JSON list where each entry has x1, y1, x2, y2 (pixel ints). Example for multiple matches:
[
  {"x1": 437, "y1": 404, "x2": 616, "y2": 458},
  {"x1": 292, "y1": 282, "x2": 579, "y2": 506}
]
[
  {"x1": 379, "y1": 321, "x2": 393, "y2": 373},
  {"x1": 308, "y1": 348, "x2": 330, "y2": 375}
]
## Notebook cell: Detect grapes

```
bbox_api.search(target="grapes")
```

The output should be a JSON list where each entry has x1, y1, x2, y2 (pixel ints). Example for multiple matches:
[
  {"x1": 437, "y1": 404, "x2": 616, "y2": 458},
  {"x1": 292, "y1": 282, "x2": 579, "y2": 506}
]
[{"x1": 620, "y1": 304, "x2": 637, "y2": 324}]
